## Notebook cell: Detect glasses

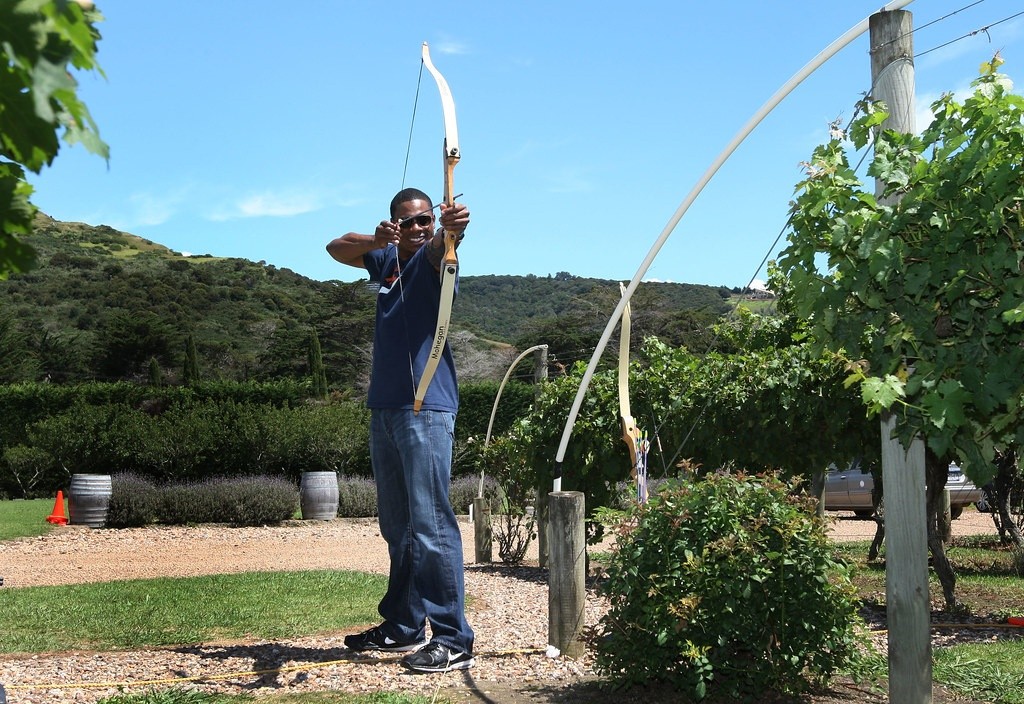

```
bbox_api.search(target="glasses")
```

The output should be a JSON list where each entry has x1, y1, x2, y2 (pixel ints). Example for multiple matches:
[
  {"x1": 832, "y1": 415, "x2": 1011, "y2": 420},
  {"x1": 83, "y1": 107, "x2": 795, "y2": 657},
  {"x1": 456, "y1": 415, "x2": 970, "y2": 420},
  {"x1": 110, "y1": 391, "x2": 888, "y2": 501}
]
[{"x1": 394, "y1": 215, "x2": 435, "y2": 229}]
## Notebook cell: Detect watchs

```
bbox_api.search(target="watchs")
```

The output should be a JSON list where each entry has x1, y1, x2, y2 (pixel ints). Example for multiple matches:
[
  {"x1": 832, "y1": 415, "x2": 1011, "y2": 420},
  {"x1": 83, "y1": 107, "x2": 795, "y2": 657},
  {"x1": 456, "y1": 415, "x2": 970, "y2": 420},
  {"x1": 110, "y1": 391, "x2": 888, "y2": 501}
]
[{"x1": 442, "y1": 228, "x2": 465, "y2": 242}]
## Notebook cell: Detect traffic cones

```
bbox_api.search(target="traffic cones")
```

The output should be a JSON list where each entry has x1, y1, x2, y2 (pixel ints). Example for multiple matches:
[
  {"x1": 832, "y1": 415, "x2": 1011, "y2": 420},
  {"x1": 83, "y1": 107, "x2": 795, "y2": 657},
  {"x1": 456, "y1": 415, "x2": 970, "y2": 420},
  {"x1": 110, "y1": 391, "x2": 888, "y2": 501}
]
[{"x1": 46, "y1": 490, "x2": 68, "y2": 524}]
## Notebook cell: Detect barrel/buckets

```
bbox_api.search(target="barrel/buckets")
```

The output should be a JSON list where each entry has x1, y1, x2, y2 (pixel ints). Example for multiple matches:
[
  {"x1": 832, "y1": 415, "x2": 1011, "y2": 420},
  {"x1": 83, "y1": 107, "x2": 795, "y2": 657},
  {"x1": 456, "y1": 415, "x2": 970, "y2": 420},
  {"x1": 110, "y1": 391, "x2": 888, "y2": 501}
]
[
  {"x1": 68, "y1": 473, "x2": 112, "y2": 529},
  {"x1": 301, "y1": 471, "x2": 340, "y2": 522}
]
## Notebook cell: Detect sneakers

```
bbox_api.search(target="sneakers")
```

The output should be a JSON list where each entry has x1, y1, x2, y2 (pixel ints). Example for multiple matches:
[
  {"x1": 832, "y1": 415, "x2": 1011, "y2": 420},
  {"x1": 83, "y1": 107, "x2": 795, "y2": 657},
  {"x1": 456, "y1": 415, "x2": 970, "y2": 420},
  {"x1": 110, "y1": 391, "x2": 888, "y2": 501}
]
[
  {"x1": 400, "y1": 643, "x2": 476, "y2": 672},
  {"x1": 343, "y1": 620, "x2": 426, "y2": 651}
]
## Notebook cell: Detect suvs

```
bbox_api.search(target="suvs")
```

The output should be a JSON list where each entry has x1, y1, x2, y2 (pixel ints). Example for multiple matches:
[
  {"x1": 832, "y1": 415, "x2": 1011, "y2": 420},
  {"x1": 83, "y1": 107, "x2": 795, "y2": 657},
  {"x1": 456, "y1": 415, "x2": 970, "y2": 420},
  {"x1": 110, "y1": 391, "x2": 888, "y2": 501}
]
[{"x1": 785, "y1": 460, "x2": 982, "y2": 519}]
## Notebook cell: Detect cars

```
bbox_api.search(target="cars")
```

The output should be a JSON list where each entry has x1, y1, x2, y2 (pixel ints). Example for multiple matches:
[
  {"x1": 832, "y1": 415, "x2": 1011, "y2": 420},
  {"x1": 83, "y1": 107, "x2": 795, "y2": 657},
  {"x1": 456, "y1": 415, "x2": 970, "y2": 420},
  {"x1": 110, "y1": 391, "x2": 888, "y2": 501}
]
[{"x1": 973, "y1": 489, "x2": 994, "y2": 513}]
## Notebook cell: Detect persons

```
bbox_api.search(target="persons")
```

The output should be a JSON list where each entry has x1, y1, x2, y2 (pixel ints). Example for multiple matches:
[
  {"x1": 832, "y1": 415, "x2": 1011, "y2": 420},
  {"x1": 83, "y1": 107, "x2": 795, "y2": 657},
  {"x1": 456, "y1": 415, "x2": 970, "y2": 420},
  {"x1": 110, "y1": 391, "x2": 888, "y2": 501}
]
[{"x1": 325, "y1": 188, "x2": 478, "y2": 674}]
